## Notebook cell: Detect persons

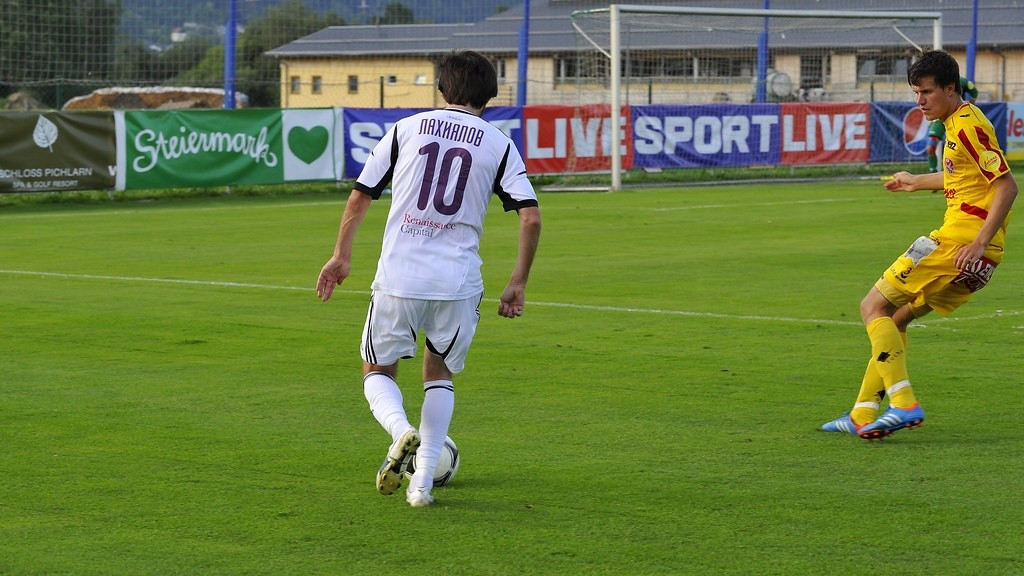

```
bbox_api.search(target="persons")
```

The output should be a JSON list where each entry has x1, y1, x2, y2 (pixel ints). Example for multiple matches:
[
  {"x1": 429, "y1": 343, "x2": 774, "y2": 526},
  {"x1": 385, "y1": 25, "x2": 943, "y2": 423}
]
[
  {"x1": 927, "y1": 77, "x2": 978, "y2": 191},
  {"x1": 820, "y1": 50, "x2": 1019, "y2": 443},
  {"x1": 317, "y1": 48, "x2": 541, "y2": 506}
]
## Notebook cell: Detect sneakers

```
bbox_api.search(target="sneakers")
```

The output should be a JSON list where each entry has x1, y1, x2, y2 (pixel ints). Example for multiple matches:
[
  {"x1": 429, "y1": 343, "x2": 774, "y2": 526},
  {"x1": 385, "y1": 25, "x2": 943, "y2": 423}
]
[
  {"x1": 822, "y1": 411, "x2": 874, "y2": 435},
  {"x1": 376, "y1": 426, "x2": 421, "y2": 495},
  {"x1": 405, "y1": 486, "x2": 434, "y2": 507},
  {"x1": 858, "y1": 400, "x2": 923, "y2": 441}
]
